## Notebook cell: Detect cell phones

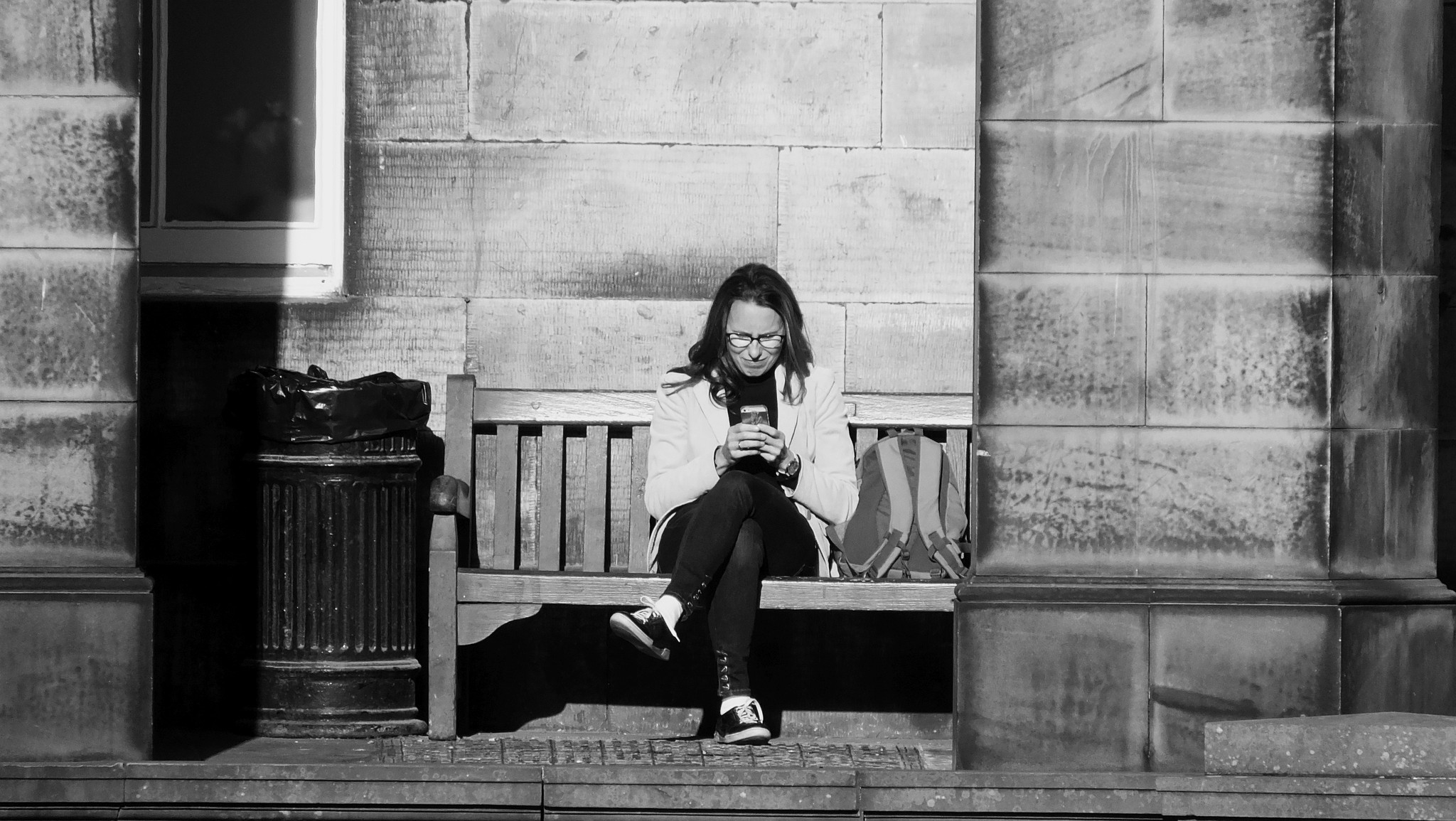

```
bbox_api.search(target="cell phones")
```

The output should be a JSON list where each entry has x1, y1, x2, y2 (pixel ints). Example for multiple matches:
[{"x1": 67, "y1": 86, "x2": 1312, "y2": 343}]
[{"x1": 740, "y1": 405, "x2": 770, "y2": 452}]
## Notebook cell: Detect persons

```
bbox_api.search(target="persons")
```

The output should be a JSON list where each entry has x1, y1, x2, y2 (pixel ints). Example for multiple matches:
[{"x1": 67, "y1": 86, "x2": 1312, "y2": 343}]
[{"x1": 609, "y1": 262, "x2": 860, "y2": 745}]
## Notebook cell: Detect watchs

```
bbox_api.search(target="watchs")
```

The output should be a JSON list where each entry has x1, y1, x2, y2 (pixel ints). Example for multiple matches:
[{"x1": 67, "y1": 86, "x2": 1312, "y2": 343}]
[{"x1": 776, "y1": 449, "x2": 800, "y2": 477}]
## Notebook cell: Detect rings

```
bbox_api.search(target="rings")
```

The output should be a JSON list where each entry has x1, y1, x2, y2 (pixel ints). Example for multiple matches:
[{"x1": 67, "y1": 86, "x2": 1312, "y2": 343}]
[{"x1": 738, "y1": 440, "x2": 743, "y2": 450}]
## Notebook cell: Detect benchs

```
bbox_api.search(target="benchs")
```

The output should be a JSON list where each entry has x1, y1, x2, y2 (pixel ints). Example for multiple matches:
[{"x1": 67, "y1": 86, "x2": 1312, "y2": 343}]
[{"x1": 428, "y1": 374, "x2": 973, "y2": 740}]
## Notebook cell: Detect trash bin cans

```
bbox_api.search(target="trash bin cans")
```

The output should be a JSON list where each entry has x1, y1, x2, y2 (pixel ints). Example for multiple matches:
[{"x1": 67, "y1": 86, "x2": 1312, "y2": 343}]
[{"x1": 234, "y1": 370, "x2": 432, "y2": 742}]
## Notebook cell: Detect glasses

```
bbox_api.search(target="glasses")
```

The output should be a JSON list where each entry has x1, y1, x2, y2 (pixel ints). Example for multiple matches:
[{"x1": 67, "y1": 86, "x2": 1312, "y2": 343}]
[{"x1": 724, "y1": 333, "x2": 786, "y2": 349}]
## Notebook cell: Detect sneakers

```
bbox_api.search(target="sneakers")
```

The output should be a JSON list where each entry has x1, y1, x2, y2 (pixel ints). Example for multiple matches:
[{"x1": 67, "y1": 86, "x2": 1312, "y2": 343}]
[
  {"x1": 610, "y1": 596, "x2": 681, "y2": 661},
  {"x1": 714, "y1": 698, "x2": 771, "y2": 743}
]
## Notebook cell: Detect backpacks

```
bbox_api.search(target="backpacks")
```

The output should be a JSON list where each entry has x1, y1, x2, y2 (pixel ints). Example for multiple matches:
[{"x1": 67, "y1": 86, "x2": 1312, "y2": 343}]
[{"x1": 824, "y1": 428, "x2": 971, "y2": 579}]
